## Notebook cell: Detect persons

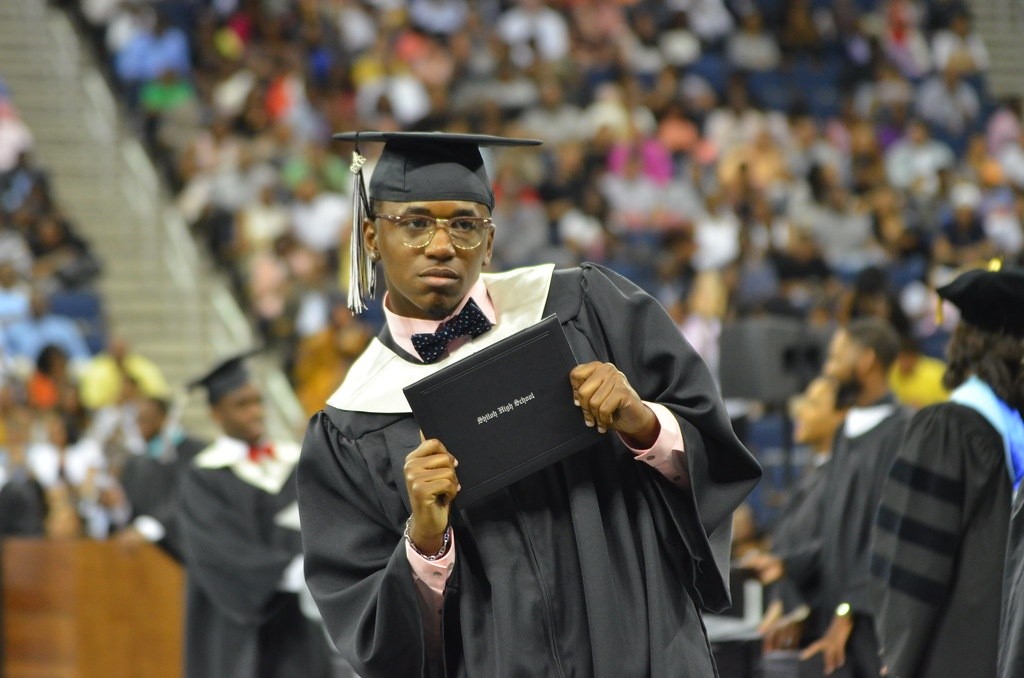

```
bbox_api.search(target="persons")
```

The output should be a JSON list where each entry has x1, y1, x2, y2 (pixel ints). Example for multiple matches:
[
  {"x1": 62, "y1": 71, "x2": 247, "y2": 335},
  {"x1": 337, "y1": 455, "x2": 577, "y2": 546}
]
[
  {"x1": 296, "y1": 130, "x2": 763, "y2": 678},
  {"x1": 48, "y1": 1, "x2": 1024, "y2": 678},
  {"x1": 0, "y1": 92, "x2": 361, "y2": 678}
]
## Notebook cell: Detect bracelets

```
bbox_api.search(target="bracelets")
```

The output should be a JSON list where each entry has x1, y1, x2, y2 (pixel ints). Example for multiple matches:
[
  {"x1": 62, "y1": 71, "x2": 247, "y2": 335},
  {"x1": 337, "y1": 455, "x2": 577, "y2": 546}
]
[{"x1": 400, "y1": 513, "x2": 450, "y2": 560}]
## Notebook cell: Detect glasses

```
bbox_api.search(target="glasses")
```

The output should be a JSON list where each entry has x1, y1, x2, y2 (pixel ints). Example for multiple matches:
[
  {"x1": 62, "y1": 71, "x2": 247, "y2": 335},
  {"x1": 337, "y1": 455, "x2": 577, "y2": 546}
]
[{"x1": 369, "y1": 214, "x2": 490, "y2": 250}]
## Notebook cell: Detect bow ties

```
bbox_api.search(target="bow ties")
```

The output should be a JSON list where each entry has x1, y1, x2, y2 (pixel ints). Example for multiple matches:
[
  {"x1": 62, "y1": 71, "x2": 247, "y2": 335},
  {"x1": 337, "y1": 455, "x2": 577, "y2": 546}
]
[
  {"x1": 413, "y1": 299, "x2": 492, "y2": 362},
  {"x1": 249, "y1": 444, "x2": 274, "y2": 461}
]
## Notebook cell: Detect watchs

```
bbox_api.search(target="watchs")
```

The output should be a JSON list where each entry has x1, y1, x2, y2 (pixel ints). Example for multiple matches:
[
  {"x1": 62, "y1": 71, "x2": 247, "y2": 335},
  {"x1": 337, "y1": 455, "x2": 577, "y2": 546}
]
[{"x1": 837, "y1": 603, "x2": 850, "y2": 616}]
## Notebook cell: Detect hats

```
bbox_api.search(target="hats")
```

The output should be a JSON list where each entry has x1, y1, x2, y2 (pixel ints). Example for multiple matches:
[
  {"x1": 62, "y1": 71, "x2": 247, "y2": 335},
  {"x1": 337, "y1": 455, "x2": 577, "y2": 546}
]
[
  {"x1": 186, "y1": 342, "x2": 266, "y2": 408},
  {"x1": 331, "y1": 130, "x2": 544, "y2": 317},
  {"x1": 936, "y1": 267, "x2": 1023, "y2": 338}
]
